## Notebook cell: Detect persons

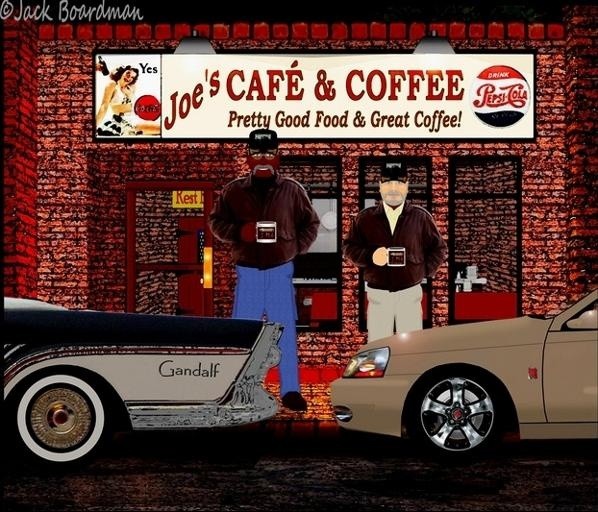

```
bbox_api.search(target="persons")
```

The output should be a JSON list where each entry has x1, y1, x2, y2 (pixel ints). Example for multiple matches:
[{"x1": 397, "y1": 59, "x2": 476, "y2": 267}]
[
  {"x1": 341, "y1": 158, "x2": 445, "y2": 345},
  {"x1": 206, "y1": 129, "x2": 320, "y2": 411}
]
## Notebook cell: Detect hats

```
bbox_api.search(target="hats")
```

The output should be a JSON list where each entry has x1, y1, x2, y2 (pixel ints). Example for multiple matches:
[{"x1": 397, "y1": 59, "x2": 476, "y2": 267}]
[
  {"x1": 379, "y1": 158, "x2": 408, "y2": 177},
  {"x1": 247, "y1": 129, "x2": 278, "y2": 150}
]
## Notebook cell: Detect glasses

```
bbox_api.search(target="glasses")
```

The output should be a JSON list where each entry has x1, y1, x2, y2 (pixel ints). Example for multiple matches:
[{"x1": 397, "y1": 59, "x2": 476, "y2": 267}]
[{"x1": 249, "y1": 151, "x2": 275, "y2": 161}]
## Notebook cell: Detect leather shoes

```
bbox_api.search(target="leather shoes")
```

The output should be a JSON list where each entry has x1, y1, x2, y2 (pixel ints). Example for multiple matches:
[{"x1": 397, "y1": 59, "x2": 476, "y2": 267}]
[{"x1": 281, "y1": 391, "x2": 307, "y2": 414}]
